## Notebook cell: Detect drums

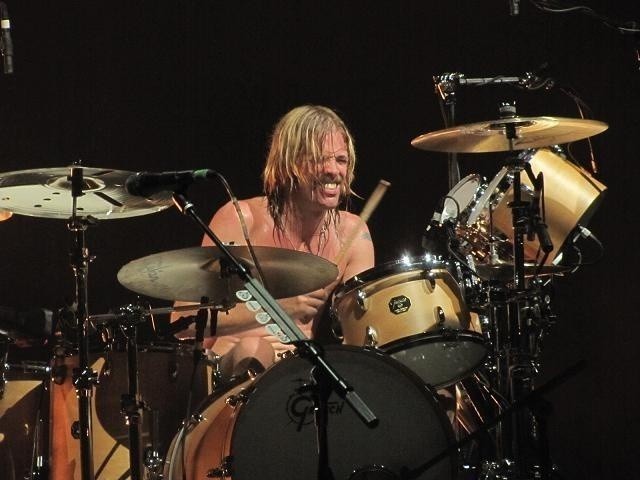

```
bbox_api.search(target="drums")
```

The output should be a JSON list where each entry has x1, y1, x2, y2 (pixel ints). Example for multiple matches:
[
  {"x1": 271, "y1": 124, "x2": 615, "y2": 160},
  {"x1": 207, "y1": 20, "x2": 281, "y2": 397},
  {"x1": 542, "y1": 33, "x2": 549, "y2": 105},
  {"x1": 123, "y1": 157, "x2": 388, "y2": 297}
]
[
  {"x1": 163, "y1": 344, "x2": 458, "y2": 480},
  {"x1": 50, "y1": 341, "x2": 221, "y2": 480},
  {"x1": 0, "y1": 357, "x2": 51, "y2": 480},
  {"x1": 467, "y1": 147, "x2": 607, "y2": 266},
  {"x1": 438, "y1": 174, "x2": 511, "y2": 260},
  {"x1": 325, "y1": 255, "x2": 490, "y2": 391}
]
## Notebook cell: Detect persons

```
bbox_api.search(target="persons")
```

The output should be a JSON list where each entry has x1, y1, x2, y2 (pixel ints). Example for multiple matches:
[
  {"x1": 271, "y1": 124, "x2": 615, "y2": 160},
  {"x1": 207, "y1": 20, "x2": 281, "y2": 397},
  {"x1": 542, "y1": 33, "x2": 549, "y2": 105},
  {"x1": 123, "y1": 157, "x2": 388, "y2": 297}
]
[{"x1": 171, "y1": 105, "x2": 376, "y2": 377}]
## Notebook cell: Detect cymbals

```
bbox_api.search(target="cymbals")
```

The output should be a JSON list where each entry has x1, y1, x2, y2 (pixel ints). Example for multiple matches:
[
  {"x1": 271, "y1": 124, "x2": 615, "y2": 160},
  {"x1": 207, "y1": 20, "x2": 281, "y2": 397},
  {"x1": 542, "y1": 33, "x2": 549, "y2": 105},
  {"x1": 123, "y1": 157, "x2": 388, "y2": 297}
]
[
  {"x1": 117, "y1": 246, "x2": 338, "y2": 302},
  {"x1": 0, "y1": 166, "x2": 175, "y2": 220},
  {"x1": 411, "y1": 116, "x2": 609, "y2": 153}
]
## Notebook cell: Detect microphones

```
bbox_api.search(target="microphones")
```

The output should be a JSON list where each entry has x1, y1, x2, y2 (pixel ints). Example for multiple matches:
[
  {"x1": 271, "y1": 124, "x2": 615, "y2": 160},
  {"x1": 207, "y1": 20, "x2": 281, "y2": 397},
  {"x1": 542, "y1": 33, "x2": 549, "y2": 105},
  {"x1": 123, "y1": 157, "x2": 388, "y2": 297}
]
[
  {"x1": 125, "y1": 168, "x2": 212, "y2": 196},
  {"x1": 420, "y1": 197, "x2": 446, "y2": 250},
  {"x1": 192, "y1": 295, "x2": 210, "y2": 369},
  {"x1": 508, "y1": 0, "x2": 520, "y2": 15},
  {"x1": 526, "y1": 171, "x2": 543, "y2": 240}
]
